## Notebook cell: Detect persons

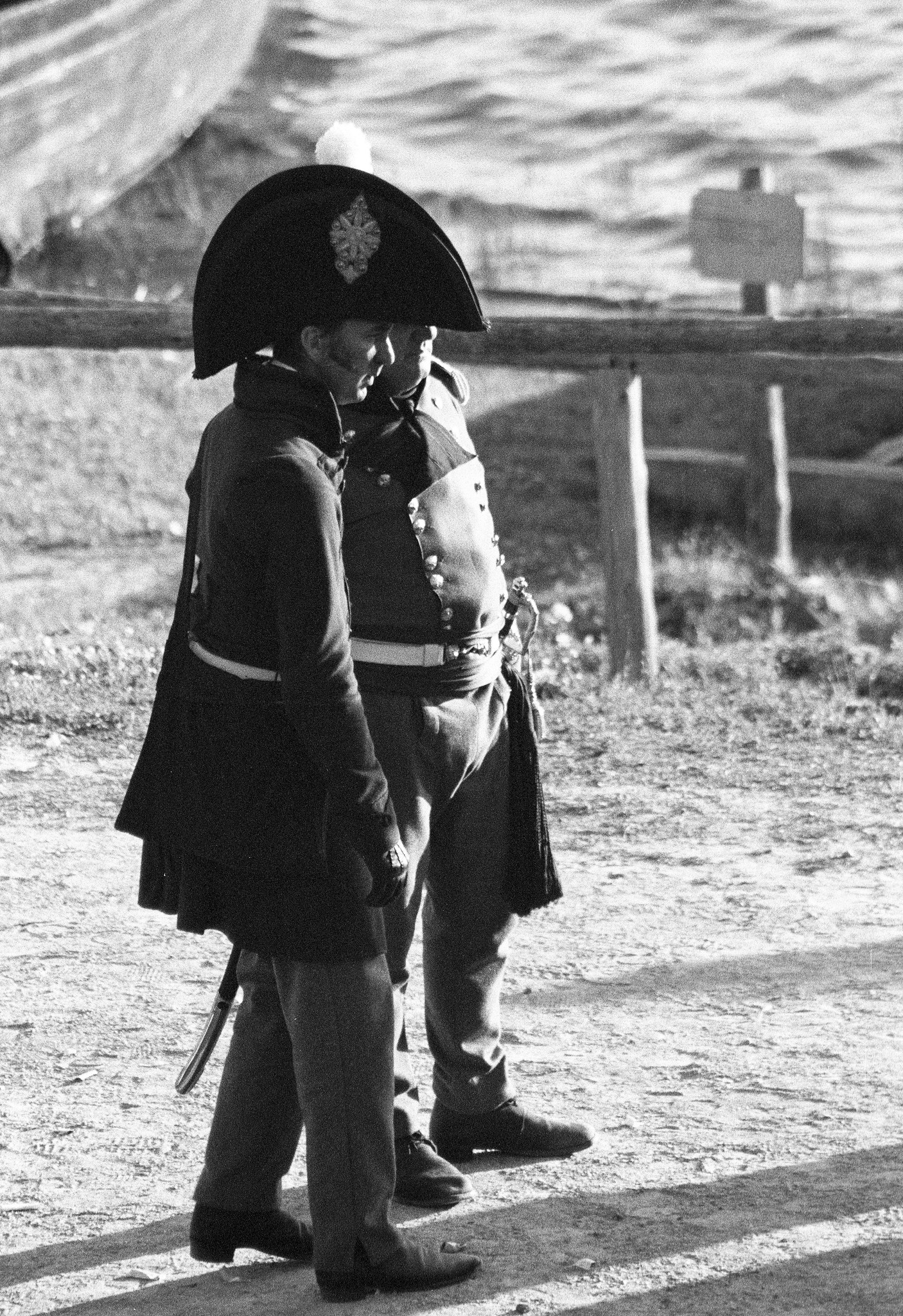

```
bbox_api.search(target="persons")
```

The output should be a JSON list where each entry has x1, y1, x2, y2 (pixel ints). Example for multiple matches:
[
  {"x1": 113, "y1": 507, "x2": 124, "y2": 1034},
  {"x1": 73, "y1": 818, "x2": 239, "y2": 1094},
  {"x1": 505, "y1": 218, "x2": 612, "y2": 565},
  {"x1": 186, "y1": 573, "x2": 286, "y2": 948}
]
[
  {"x1": 110, "y1": 166, "x2": 491, "y2": 1305},
  {"x1": 341, "y1": 325, "x2": 597, "y2": 1210}
]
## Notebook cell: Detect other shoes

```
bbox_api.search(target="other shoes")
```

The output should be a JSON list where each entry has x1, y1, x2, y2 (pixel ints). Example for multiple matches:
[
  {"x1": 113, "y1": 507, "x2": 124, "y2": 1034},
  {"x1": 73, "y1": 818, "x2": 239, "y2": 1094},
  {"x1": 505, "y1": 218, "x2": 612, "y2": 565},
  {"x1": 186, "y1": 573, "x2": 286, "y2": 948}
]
[
  {"x1": 313, "y1": 1241, "x2": 483, "y2": 1304},
  {"x1": 429, "y1": 1096, "x2": 594, "y2": 1164},
  {"x1": 392, "y1": 1130, "x2": 478, "y2": 1204},
  {"x1": 188, "y1": 1200, "x2": 315, "y2": 1263}
]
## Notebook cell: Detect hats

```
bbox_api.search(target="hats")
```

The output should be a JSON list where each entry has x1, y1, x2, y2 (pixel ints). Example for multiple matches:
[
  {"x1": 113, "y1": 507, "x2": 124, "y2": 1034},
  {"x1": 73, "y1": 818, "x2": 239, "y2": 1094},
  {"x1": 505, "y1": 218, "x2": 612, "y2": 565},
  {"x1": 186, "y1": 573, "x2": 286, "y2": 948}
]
[{"x1": 192, "y1": 166, "x2": 488, "y2": 380}]
